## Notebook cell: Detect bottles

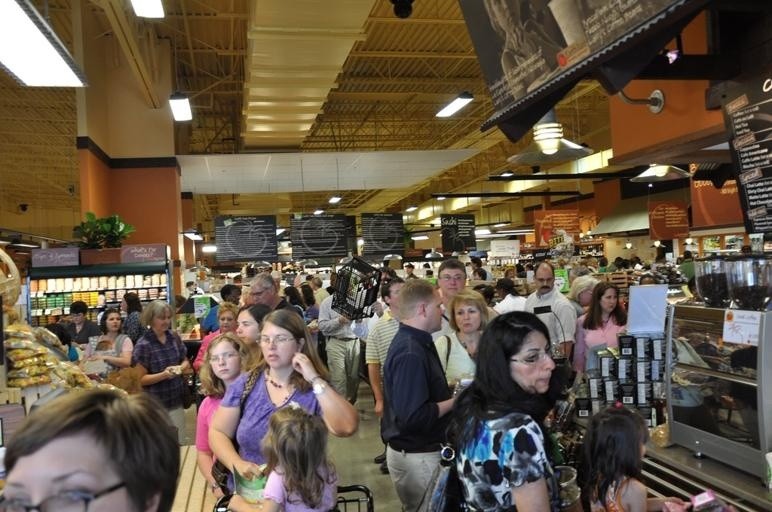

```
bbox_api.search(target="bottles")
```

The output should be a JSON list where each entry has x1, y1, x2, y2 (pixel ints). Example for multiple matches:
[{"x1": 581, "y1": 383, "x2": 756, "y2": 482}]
[{"x1": 453, "y1": 379, "x2": 474, "y2": 398}]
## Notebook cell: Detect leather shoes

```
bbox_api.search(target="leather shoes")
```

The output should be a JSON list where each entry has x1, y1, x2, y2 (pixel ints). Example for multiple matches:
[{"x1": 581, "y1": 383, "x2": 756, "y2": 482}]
[{"x1": 375, "y1": 453, "x2": 388, "y2": 471}]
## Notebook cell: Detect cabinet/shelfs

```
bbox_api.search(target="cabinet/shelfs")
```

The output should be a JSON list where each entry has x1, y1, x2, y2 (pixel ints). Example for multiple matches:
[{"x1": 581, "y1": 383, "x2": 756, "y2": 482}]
[
  {"x1": 665, "y1": 303, "x2": 772, "y2": 478},
  {"x1": 26, "y1": 243, "x2": 171, "y2": 327},
  {"x1": 520, "y1": 239, "x2": 607, "y2": 267}
]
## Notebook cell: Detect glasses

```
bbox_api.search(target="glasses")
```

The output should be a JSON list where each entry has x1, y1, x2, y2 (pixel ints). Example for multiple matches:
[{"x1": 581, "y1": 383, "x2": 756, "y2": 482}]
[
  {"x1": 439, "y1": 274, "x2": 466, "y2": 280},
  {"x1": 256, "y1": 335, "x2": 295, "y2": 346},
  {"x1": 0, "y1": 483, "x2": 124, "y2": 512},
  {"x1": 508, "y1": 346, "x2": 556, "y2": 365},
  {"x1": 249, "y1": 289, "x2": 267, "y2": 296}
]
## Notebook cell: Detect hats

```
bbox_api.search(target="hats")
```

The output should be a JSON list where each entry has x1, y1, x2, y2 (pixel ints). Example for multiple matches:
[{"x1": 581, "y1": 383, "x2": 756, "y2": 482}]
[{"x1": 405, "y1": 264, "x2": 414, "y2": 268}]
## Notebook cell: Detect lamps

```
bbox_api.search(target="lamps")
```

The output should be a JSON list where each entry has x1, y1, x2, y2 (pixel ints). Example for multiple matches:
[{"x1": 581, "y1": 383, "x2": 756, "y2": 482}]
[
  {"x1": 435, "y1": 91, "x2": 474, "y2": 118},
  {"x1": 0, "y1": 0, "x2": 89, "y2": 87},
  {"x1": 169, "y1": 92, "x2": 193, "y2": 121},
  {"x1": 533, "y1": 107, "x2": 564, "y2": 155},
  {"x1": 180, "y1": 230, "x2": 204, "y2": 241},
  {"x1": 622, "y1": 238, "x2": 637, "y2": 250},
  {"x1": 630, "y1": 161, "x2": 693, "y2": 183},
  {"x1": 500, "y1": 170, "x2": 514, "y2": 177},
  {"x1": 650, "y1": 240, "x2": 666, "y2": 248},
  {"x1": 296, "y1": 159, "x2": 469, "y2": 267},
  {"x1": 130, "y1": 0, "x2": 166, "y2": 19}
]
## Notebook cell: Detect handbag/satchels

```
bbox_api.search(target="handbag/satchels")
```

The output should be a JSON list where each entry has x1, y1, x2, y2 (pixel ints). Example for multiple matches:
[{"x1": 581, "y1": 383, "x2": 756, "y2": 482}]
[
  {"x1": 414, "y1": 460, "x2": 459, "y2": 512},
  {"x1": 183, "y1": 384, "x2": 193, "y2": 409},
  {"x1": 210, "y1": 439, "x2": 238, "y2": 490}
]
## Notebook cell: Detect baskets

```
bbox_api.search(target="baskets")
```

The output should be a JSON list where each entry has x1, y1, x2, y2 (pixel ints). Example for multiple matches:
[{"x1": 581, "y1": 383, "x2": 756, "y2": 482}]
[
  {"x1": 331, "y1": 256, "x2": 382, "y2": 320},
  {"x1": 0, "y1": 247, "x2": 21, "y2": 305}
]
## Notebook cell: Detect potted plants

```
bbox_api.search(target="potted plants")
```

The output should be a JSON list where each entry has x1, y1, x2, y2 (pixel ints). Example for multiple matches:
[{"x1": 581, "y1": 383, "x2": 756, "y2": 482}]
[
  {"x1": 72, "y1": 211, "x2": 136, "y2": 264},
  {"x1": 177, "y1": 313, "x2": 197, "y2": 339}
]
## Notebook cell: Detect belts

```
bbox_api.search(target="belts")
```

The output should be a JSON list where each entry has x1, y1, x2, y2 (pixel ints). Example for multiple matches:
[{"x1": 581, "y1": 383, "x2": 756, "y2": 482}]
[
  {"x1": 332, "y1": 336, "x2": 359, "y2": 342},
  {"x1": 387, "y1": 442, "x2": 439, "y2": 453}
]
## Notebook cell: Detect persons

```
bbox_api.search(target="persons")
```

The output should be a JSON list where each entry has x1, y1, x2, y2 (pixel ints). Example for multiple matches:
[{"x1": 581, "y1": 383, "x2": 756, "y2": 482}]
[
  {"x1": 1, "y1": 246, "x2": 771, "y2": 512},
  {"x1": 483, "y1": 0, "x2": 564, "y2": 100}
]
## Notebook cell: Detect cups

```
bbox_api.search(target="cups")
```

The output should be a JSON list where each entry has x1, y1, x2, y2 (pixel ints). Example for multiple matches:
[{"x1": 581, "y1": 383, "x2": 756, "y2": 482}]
[
  {"x1": 127, "y1": 288, "x2": 159, "y2": 300},
  {"x1": 553, "y1": 463, "x2": 580, "y2": 509},
  {"x1": 693, "y1": 252, "x2": 771, "y2": 310},
  {"x1": 487, "y1": 258, "x2": 519, "y2": 266}
]
